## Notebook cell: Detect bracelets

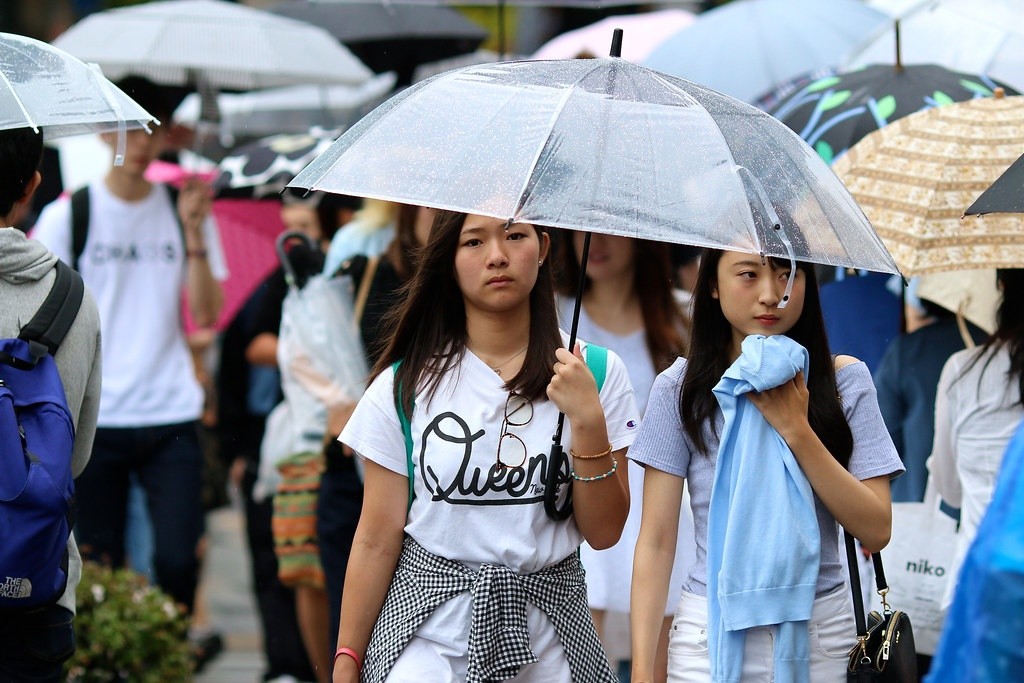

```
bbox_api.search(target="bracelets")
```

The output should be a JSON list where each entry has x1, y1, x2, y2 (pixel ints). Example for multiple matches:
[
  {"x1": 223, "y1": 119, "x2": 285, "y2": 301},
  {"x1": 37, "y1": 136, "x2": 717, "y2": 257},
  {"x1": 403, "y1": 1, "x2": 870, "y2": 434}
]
[
  {"x1": 186, "y1": 250, "x2": 207, "y2": 259},
  {"x1": 332, "y1": 647, "x2": 361, "y2": 673},
  {"x1": 570, "y1": 457, "x2": 617, "y2": 481},
  {"x1": 570, "y1": 443, "x2": 613, "y2": 459}
]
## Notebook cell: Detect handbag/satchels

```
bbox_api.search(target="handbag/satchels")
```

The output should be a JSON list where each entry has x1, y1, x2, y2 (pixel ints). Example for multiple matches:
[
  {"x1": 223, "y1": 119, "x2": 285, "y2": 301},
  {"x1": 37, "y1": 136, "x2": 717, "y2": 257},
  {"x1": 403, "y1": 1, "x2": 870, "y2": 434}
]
[
  {"x1": 254, "y1": 229, "x2": 370, "y2": 499},
  {"x1": 843, "y1": 607, "x2": 919, "y2": 683}
]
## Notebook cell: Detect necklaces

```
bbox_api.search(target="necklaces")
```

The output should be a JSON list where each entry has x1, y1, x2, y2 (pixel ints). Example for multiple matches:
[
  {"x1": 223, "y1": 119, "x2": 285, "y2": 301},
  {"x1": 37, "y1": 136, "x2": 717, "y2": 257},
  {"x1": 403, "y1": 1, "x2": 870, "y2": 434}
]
[{"x1": 488, "y1": 344, "x2": 530, "y2": 375}]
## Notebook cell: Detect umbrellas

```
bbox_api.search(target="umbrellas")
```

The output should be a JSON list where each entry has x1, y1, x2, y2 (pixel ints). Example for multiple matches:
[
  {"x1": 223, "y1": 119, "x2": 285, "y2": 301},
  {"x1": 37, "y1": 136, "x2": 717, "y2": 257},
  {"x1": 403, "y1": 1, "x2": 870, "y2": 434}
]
[
  {"x1": 268, "y1": 1, "x2": 489, "y2": 73},
  {"x1": 50, "y1": 0, "x2": 374, "y2": 202},
  {"x1": 213, "y1": 129, "x2": 349, "y2": 199},
  {"x1": 172, "y1": 73, "x2": 391, "y2": 139},
  {"x1": 532, "y1": 1, "x2": 1024, "y2": 332},
  {"x1": 1, "y1": 33, "x2": 159, "y2": 165},
  {"x1": 282, "y1": 25, "x2": 907, "y2": 522}
]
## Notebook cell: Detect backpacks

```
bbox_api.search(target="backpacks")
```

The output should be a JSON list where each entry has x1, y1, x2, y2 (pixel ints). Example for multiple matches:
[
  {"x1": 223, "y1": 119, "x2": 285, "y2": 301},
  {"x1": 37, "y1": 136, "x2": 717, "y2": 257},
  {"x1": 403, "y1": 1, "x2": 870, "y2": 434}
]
[{"x1": 0, "y1": 257, "x2": 86, "y2": 618}]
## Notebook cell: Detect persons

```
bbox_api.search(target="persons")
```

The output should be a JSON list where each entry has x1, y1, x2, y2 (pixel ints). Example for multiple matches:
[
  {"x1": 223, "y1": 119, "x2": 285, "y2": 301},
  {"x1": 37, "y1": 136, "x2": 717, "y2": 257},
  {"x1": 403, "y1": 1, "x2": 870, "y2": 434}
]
[
  {"x1": 279, "y1": 203, "x2": 325, "y2": 244},
  {"x1": 627, "y1": 201, "x2": 905, "y2": 683},
  {"x1": 0, "y1": 127, "x2": 104, "y2": 683},
  {"x1": 869, "y1": 277, "x2": 990, "y2": 504},
  {"x1": 319, "y1": 200, "x2": 440, "y2": 683},
  {"x1": 325, "y1": 177, "x2": 640, "y2": 683},
  {"x1": 817, "y1": 267, "x2": 902, "y2": 376},
  {"x1": 547, "y1": 229, "x2": 697, "y2": 682},
  {"x1": 272, "y1": 194, "x2": 399, "y2": 683},
  {"x1": 929, "y1": 270, "x2": 1024, "y2": 618},
  {"x1": 34, "y1": 76, "x2": 221, "y2": 642},
  {"x1": 217, "y1": 193, "x2": 363, "y2": 682},
  {"x1": 122, "y1": 327, "x2": 226, "y2": 677}
]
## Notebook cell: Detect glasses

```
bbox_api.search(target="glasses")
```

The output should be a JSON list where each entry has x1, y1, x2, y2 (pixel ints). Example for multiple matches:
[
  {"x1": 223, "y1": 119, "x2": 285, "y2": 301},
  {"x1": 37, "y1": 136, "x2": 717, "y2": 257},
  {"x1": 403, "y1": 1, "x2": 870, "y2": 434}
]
[{"x1": 491, "y1": 391, "x2": 535, "y2": 469}]
